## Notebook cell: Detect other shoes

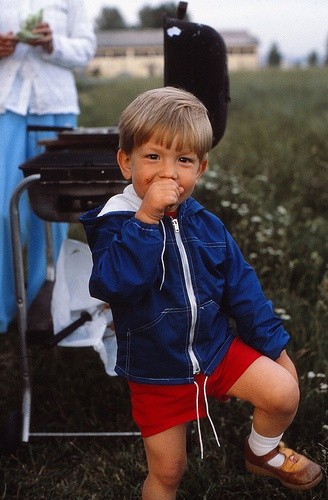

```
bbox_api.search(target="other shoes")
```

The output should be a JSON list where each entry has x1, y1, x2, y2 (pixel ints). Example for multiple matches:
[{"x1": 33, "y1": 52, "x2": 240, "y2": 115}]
[{"x1": 245, "y1": 433, "x2": 322, "y2": 491}]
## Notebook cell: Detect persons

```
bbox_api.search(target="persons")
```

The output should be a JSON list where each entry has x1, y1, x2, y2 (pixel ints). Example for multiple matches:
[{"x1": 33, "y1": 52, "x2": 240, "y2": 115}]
[
  {"x1": 0, "y1": 0, "x2": 97, "y2": 333},
  {"x1": 79, "y1": 86, "x2": 323, "y2": 500}
]
